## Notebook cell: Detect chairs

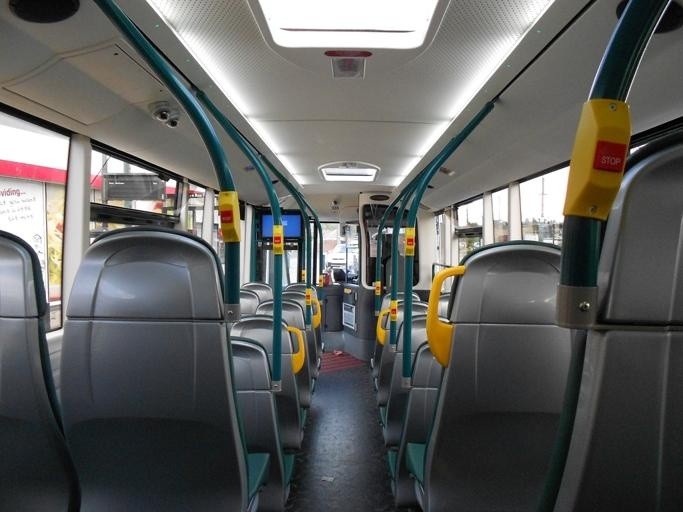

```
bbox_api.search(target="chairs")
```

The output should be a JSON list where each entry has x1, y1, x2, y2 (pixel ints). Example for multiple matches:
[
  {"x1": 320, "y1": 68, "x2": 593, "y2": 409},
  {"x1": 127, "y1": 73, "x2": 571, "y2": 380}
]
[
  {"x1": 371, "y1": 290, "x2": 451, "y2": 512},
  {"x1": 545, "y1": 114, "x2": 683, "y2": 511},
  {"x1": 228, "y1": 281, "x2": 323, "y2": 512},
  {"x1": 0, "y1": 232, "x2": 75, "y2": 511},
  {"x1": 426, "y1": 245, "x2": 583, "y2": 508},
  {"x1": 61, "y1": 226, "x2": 270, "y2": 512}
]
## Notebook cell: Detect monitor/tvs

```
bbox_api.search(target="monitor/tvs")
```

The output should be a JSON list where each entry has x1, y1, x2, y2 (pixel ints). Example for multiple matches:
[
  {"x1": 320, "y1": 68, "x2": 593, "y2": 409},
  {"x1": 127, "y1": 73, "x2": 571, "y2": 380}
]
[{"x1": 260, "y1": 209, "x2": 303, "y2": 240}]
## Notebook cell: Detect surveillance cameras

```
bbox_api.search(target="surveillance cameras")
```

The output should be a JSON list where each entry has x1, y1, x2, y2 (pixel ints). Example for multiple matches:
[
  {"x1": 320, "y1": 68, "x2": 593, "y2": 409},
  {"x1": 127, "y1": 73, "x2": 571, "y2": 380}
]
[
  {"x1": 332, "y1": 199, "x2": 338, "y2": 206},
  {"x1": 151, "y1": 102, "x2": 169, "y2": 120},
  {"x1": 162, "y1": 110, "x2": 180, "y2": 128}
]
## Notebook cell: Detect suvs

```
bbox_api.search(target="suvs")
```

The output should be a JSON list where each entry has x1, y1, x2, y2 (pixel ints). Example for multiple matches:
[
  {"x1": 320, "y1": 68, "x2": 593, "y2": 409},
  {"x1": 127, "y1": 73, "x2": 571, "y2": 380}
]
[{"x1": 324, "y1": 242, "x2": 358, "y2": 271}]
